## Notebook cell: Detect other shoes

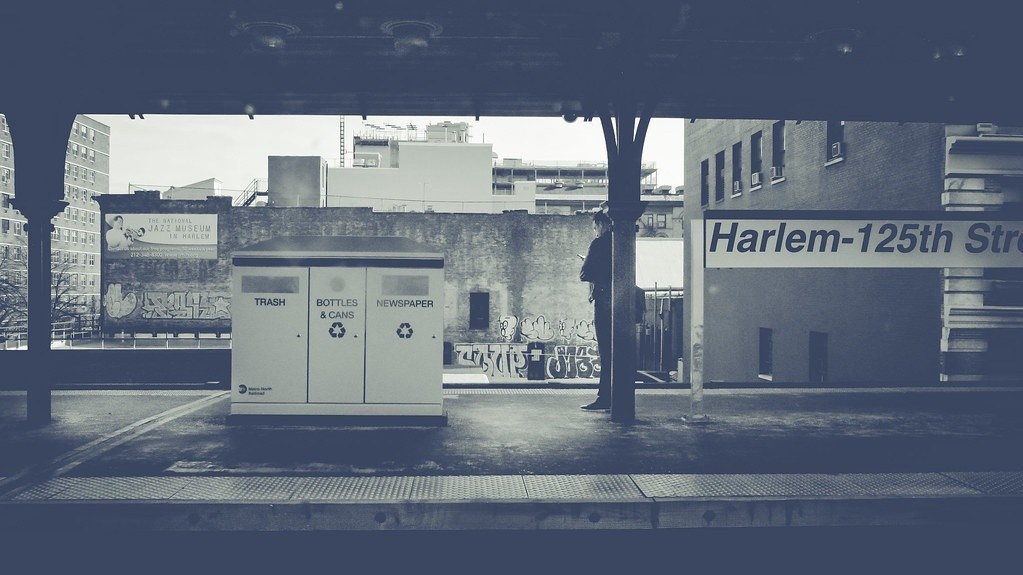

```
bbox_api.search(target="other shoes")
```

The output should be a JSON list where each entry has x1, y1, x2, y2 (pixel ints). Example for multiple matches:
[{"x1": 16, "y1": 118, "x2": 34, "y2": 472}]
[{"x1": 580, "y1": 397, "x2": 609, "y2": 412}]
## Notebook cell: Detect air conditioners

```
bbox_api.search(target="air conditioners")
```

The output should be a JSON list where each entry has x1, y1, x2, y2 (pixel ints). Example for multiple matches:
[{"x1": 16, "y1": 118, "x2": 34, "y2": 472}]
[
  {"x1": 831, "y1": 142, "x2": 843, "y2": 158},
  {"x1": 734, "y1": 181, "x2": 743, "y2": 193},
  {"x1": 751, "y1": 173, "x2": 764, "y2": 185},
  {"x1": 769, "y1": 166, "x2": 785, "y2": 179}
]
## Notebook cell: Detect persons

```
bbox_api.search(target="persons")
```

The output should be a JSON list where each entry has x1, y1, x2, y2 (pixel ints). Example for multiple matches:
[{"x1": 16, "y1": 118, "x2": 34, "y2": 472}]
[
  {"x1": 580, "y1": 210, "x2": 612, "y2": 412},
  {"x1": 106, "y1": 215, "x2": 133, "y2": 251}
]
